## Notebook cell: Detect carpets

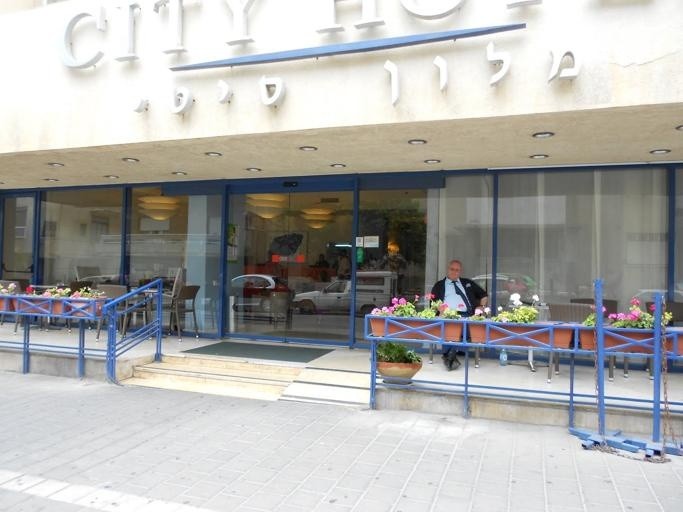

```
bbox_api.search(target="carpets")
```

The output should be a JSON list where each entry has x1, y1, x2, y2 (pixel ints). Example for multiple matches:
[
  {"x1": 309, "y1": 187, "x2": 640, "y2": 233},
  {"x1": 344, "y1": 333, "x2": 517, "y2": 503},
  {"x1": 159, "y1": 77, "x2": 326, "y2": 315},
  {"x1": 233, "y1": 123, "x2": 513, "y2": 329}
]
[{"x1": 180, "y1": 341, "x2": 336, "y2": 364}]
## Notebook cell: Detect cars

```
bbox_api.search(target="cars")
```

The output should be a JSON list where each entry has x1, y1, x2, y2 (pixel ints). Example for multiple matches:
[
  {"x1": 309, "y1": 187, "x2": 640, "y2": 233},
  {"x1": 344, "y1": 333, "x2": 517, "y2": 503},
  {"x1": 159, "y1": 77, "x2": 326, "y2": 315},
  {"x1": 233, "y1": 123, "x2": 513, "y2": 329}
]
[
  {"x1": 230, "y1": 269, "x2": 400, "y2": 316},
  {"x1": 470, "y1": 272, "x2": 683, "y2": 313}
]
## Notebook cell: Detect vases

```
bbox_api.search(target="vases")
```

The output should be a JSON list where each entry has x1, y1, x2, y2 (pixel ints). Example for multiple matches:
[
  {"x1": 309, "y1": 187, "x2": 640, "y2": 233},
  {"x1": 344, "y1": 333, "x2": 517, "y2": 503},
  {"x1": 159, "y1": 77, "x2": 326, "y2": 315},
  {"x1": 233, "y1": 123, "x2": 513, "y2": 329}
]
[
  {"x1": 0, "y1": 297, "x2": 71, "y2": 315},
  {"x1": 369, "y1": 317, "x2": 575, "y2": 356}
]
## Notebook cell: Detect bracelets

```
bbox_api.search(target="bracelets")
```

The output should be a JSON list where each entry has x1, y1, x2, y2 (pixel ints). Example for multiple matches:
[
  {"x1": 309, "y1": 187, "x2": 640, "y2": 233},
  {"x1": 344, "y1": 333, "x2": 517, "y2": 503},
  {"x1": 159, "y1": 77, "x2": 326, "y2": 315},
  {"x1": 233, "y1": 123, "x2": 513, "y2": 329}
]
[{"x1": 480, "y1": 305, "x2": 485, "y2": 307}]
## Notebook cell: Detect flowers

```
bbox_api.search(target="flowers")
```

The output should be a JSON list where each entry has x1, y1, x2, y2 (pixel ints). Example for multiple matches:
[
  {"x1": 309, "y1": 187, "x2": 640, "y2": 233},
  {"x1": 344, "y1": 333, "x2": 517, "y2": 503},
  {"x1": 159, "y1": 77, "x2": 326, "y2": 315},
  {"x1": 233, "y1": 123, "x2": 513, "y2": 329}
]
[
  {"x1": 0, "y1": 282, "x2": 72, "y2": 296},
  {"x1": 371, "y1": 291, "x2": 541, "y2": 331}
]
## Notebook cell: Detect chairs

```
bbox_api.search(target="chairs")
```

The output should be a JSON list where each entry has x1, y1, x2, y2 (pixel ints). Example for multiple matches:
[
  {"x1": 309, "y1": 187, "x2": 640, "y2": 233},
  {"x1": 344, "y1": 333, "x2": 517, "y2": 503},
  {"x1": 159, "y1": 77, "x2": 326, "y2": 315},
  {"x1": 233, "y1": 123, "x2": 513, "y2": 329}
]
[
  {"x1": 69, "y1": 263, "x2": 198, "y2": 338},
  {"x1": 548, "y1": 297, "x2": 683, "y2": 383}
]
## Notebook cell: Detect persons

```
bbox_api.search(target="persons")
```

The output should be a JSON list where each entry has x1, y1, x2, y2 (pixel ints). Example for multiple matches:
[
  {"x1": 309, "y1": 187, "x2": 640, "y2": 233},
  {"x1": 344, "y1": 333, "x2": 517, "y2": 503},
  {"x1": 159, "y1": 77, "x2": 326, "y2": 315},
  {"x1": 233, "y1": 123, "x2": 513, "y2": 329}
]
[
  {"x1": 315, "y1": 248, "x2": 352, "y2": 281},
  {"x1": 430, "y1": 260, "x2": 489, "y2": 371},
  {"x1": 377, "y1": 241, "x2": 408, "y2": 297}
]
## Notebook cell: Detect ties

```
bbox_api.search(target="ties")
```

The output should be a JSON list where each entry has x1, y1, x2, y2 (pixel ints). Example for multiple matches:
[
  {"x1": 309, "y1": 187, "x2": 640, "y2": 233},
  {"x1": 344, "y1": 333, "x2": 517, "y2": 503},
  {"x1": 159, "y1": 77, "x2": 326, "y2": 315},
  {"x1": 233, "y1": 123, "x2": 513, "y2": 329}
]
[{"x1": 451, "y1": 281, "x2": 471, "y2": 313}]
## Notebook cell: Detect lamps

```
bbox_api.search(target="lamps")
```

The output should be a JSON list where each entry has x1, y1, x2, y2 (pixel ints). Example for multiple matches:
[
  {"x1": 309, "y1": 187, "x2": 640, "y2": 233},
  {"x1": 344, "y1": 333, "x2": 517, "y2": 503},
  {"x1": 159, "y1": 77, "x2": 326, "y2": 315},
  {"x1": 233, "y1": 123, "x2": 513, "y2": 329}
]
[
  {"x1": 136, "y1": 195, "x2": 181, "y2": 221},
  {"x1": 246, "y1": 193, "x2": 288, "y2": 218},
  {"x1": 300, "y1": 207, "x2": 336, "y2": 230}
]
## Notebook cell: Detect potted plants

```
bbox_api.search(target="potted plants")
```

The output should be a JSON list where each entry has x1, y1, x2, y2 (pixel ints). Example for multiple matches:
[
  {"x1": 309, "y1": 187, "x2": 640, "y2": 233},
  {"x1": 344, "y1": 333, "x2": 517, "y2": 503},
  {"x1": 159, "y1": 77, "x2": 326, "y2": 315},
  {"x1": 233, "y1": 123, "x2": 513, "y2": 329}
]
[{"x1": 376, "y1": 341, "x2": 424, "y2": 385}]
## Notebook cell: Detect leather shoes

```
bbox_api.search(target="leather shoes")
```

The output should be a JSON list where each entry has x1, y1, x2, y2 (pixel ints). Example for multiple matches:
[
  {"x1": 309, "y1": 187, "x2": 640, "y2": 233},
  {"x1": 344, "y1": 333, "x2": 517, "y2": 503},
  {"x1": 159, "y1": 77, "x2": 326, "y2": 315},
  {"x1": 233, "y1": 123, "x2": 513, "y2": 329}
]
[{"x1": 441, "y1": 354, "x2": 454, "y2": 370}]
